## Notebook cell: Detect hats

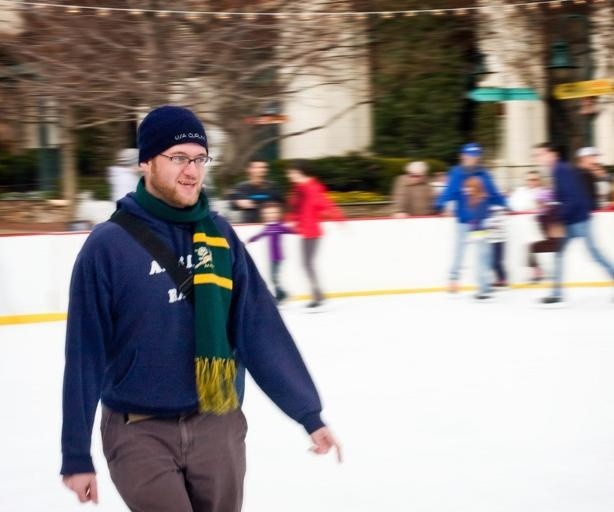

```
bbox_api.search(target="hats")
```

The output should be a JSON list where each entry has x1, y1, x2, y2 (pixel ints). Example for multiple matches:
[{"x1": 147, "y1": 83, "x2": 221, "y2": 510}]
[
  {"x1": 460, "y1": 143, "x2": 485, "y2": 158},
  {"x1": 574, "y1": 145, "x2": 602, "y2": 159}
]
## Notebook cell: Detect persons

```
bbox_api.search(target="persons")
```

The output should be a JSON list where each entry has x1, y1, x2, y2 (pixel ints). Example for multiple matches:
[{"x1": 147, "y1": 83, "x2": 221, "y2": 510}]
[
  {"x1": 218, "y1": 158, "x2": 287, "y2": 223},
  {"x1": 431, "y1": 142, "x2": 512, "y2": 294},
  {"x1": 56, "y1": 104, "x2": 343, "y2": 512},
  {"x1": 530, "y1": 139, "x2": 614, "y2": 303},
  {"x1": 502, "y1": 170, "x2": 555, "y2": 282},
  {"x1": 386, "y1": 158, "x2": 446, "y2": 217},
  {"x1": 574, "y1": 146, "x2": 613, "y2": 212},
  {"x1": 458, "y1": 173, "x2": 524, "y2": 288},
  {"x1": 239, "y1": 203, "x2": 302, "y2": 303},
  {"x1": 280, "y1": 165, "x2": 348, "y2": 309}
]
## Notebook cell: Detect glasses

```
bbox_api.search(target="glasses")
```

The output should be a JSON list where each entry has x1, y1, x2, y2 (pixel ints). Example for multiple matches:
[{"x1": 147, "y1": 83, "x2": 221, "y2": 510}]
[
  {"x1": 136, "y1": 104, "x2": 209, "y2": 162},
  {"x1": 159, "y1": 153, "x2": 214, "y2": 169}
]
